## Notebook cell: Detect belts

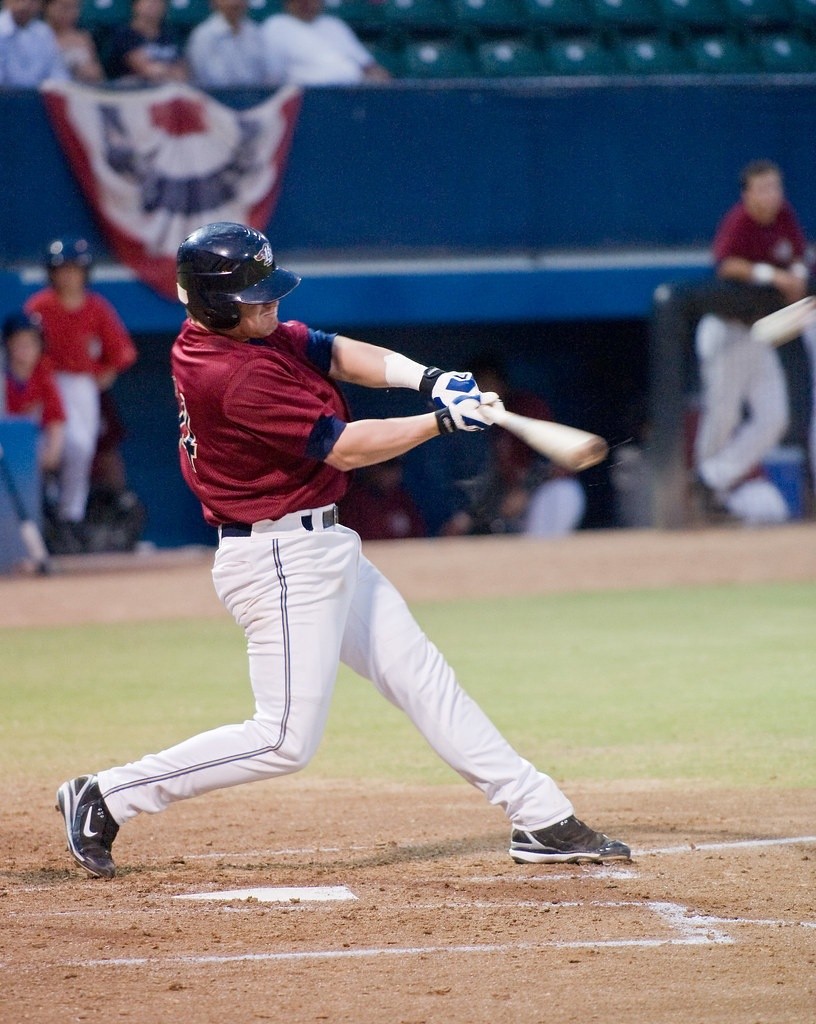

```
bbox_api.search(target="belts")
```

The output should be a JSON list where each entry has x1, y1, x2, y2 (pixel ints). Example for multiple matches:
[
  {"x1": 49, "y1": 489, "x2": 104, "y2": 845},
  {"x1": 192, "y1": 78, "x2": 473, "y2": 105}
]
[{"x1": 221, "y1": 506, "x2": 340, "y2": 537}]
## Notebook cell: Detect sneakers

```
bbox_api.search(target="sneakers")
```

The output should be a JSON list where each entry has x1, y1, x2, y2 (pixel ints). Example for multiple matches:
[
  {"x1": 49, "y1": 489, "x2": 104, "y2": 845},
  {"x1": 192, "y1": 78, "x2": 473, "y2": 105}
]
[
  {"x1": 510, "y1": 814, "x2": 631, "y2": 862},
  {"x1": 55, "y1": 774, "x2": 119, "y2": 878}
]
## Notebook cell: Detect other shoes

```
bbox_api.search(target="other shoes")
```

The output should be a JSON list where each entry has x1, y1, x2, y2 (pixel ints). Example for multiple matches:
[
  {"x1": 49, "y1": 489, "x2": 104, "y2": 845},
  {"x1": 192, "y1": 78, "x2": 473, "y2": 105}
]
[{"x1": 687, "y1": 472, "x2": 726, "y2": 517}]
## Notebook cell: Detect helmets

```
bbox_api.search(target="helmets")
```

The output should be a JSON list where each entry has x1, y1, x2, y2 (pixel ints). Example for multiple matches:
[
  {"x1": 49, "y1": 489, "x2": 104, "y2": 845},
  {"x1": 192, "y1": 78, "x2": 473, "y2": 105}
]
[
  {"x1": 176, "y1": 222, "x2": 301, "y2": 331},
  {"x1": 4, "y1": 313, "x2": 45, "y2": 338},
  {"x1": 45, "y1": 237, "x2": 96, "y2": 272}
]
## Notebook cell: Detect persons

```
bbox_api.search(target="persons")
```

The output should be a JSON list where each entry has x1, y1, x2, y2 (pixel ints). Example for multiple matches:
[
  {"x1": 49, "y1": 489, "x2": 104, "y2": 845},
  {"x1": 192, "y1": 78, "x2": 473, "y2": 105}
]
[
  {"x1": 54, "y1": 222, "x2": 631, "y2": 880},
  {"x1": 0, "y1": 0, "x2": 816, "y2": 578}
]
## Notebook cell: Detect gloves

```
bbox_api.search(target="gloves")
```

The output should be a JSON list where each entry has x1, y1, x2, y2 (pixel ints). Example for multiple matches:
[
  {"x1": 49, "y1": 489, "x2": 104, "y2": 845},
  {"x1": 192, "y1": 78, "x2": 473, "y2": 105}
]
[
  {"x1": 435, "y1": 392, "x2": 503, "y2": 434},
  {"x1": 419, "y1": 367, "x2": 479, "y2": 407}
]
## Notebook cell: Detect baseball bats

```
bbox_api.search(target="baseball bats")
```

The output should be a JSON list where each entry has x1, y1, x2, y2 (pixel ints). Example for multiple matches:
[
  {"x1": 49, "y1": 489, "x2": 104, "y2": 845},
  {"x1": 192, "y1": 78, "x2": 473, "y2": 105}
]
[{"x1": 481, "y1": 404, "x2": 610, "y2": 473}]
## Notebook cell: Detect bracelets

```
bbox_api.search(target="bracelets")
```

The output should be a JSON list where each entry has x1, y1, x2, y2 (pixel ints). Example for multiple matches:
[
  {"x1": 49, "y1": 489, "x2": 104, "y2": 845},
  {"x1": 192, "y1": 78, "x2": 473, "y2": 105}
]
[{"x1": 750, "y1": 262, "x2": 774, "y2": 285}]
